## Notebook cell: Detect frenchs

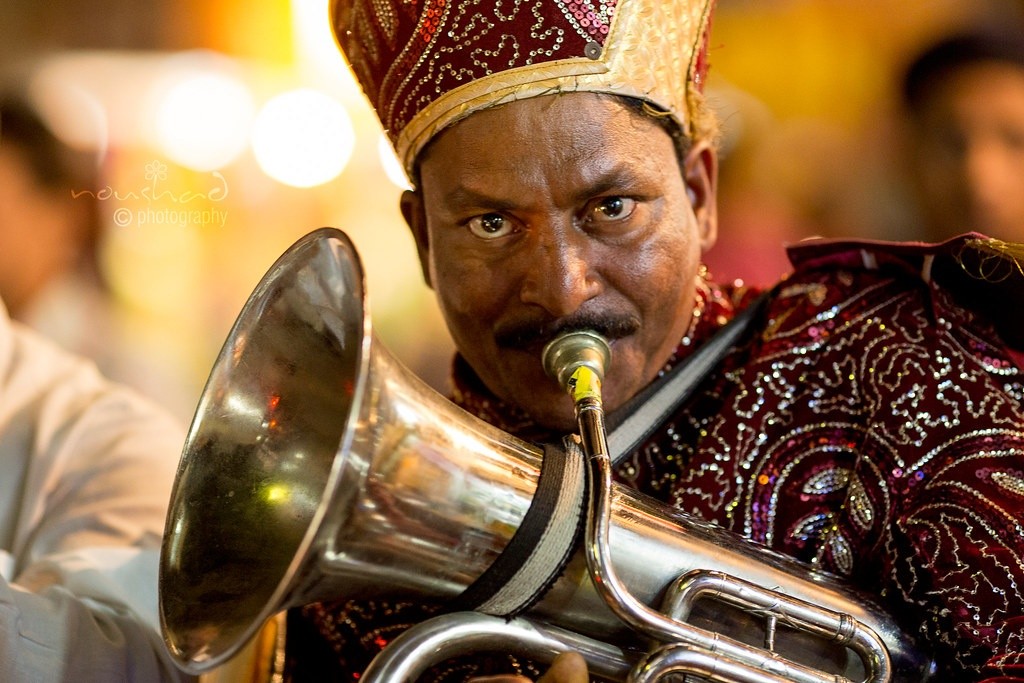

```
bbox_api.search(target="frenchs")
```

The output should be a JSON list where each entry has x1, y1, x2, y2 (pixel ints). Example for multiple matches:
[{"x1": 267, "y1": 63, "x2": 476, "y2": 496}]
[{"x1": 155, "y1": 232, "x2": 959, "y2": 683}]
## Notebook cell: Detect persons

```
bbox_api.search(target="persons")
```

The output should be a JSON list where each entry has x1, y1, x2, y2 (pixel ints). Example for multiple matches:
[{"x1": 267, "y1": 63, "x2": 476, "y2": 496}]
[
  {"x1": 0, "y1": 87, "x2": 201, "y2": 683},
  {"x1": 284, "y1": 0, "x2": 1024, "y2": 683},
  {"x1": 895, "y1": 23, "x2": 1024, "y2": 247}
]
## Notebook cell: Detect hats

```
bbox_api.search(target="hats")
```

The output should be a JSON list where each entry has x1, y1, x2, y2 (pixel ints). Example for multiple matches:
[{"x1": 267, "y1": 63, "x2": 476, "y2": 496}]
[{"x1": 328, "y1": 0, "x2": 724, "y2": 188}]
